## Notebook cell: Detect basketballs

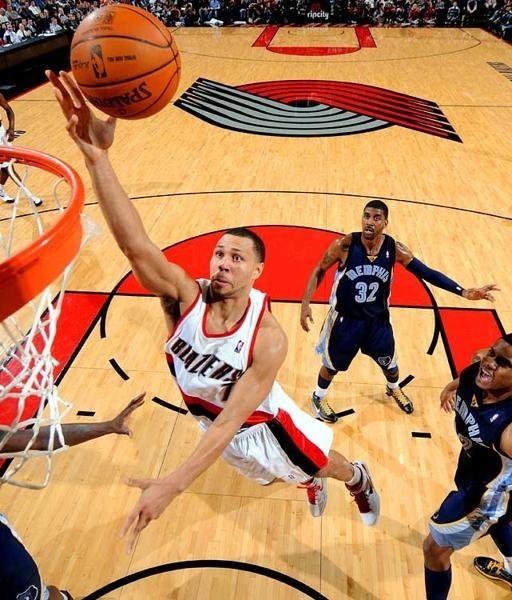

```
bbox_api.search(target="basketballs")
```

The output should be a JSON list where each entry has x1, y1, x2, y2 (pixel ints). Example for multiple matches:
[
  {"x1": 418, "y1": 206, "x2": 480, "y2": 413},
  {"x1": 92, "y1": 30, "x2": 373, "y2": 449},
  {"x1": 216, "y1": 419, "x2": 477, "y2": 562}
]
[{"x1": 70, "y1": 4, "x2": 182, "y2": 120}]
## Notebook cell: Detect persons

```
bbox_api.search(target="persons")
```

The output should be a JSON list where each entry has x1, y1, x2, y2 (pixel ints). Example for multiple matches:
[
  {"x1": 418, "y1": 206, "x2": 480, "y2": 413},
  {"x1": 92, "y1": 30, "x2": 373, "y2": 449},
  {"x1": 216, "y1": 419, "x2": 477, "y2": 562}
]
[
  {"x1": 0, "y1": 390, "x2": 149, "y2": 600},
  {"x1": 299, "y1": 196, "x2": 505, "y2": 425},
  {"x1": 419, "y1": 331, "x2": 512, "y2": 600},
  {"x1": 0, "y1": 91, "x2": 44, "y2": 208},
  {"x1": 45, "y1": 68, "x2": 382, "y2": 558}
]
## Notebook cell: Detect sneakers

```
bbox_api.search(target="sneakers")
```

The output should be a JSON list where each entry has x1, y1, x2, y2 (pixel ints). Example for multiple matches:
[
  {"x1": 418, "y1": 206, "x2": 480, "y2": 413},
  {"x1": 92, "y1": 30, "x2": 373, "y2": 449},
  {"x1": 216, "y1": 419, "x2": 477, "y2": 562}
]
[
  {"x1": 297, "y1": 475, "x2": 328, "y2": 518},
  {"x1": 473, "y1": 556, "x2": 512, "y2": 591},
  {"x1": 386, "y1": 383, "x2": 414, "y2": 414},
  {"x1": 345, "y1": 461, "x2": 380, "y2": 527},
  {"x1": 311, "y1": 390, "x2": 338, "y2": 423}
]
